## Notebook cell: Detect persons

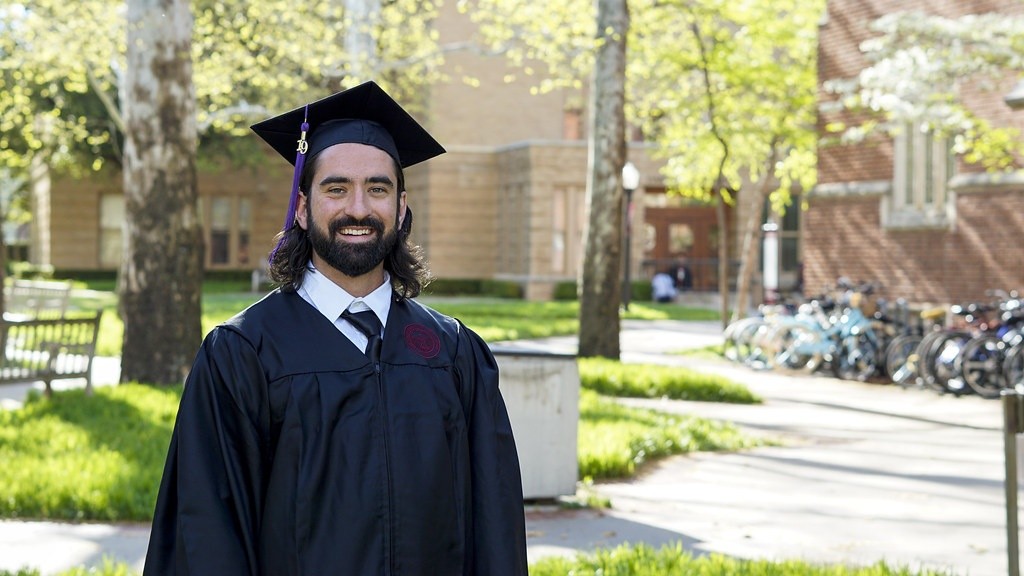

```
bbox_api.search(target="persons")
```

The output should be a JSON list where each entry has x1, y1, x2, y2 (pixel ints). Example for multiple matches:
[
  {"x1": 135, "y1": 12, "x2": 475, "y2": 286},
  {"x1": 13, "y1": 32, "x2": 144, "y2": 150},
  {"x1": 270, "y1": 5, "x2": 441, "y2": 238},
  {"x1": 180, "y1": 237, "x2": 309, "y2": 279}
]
[
  {"x1": 142, "y1": 81, "x2": 528, "y2": 576},
  {"x1": 650, "y1": 268, "x2": 675, "y2": 304},
  {"x1": 669, "y1": 251, "x2": 692, "y2": 293}
]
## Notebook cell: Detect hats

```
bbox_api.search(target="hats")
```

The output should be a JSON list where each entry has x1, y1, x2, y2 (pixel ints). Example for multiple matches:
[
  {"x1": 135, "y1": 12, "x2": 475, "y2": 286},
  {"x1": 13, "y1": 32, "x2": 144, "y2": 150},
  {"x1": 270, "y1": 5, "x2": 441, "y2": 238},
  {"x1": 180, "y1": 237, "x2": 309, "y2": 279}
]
[{"x1": 248, "y1": 81, "x2": 448, "y2": 259}]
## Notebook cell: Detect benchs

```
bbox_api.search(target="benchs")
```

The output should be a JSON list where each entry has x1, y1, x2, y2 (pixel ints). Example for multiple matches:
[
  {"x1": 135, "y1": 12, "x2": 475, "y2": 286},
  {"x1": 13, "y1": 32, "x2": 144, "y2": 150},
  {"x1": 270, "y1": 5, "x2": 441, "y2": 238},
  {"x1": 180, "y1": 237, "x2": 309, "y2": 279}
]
[
  {"x1": 0, "y1": 308, "x2": 104, "y2": 398},
  {"x1": 6, "y1": 278, "x2": 74, "y2": 336}
]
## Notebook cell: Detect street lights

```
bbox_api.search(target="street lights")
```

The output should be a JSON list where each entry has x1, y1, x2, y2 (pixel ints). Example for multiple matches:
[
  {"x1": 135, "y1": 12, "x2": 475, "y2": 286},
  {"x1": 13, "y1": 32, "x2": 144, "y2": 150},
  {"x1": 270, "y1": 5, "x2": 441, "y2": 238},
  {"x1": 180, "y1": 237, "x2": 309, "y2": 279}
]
[{"x1": 621, "y1": 157, "x2": 641, "y2": 310}]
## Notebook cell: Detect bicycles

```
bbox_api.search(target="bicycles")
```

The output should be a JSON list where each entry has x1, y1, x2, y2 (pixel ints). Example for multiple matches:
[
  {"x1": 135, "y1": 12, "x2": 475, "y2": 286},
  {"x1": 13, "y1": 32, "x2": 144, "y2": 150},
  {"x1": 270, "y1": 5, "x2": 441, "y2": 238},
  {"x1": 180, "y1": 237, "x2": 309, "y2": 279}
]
[{"x1": 719, "y1": 276, "x2": 1024, "y2": 399}]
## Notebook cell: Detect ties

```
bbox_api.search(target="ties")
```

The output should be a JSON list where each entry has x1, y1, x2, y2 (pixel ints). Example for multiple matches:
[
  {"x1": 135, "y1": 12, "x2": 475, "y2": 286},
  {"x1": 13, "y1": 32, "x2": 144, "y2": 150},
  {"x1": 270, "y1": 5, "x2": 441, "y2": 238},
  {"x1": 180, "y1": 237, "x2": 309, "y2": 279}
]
[{"x1": 339, "y1": 308, "x2": 383, "y2": 373}]
